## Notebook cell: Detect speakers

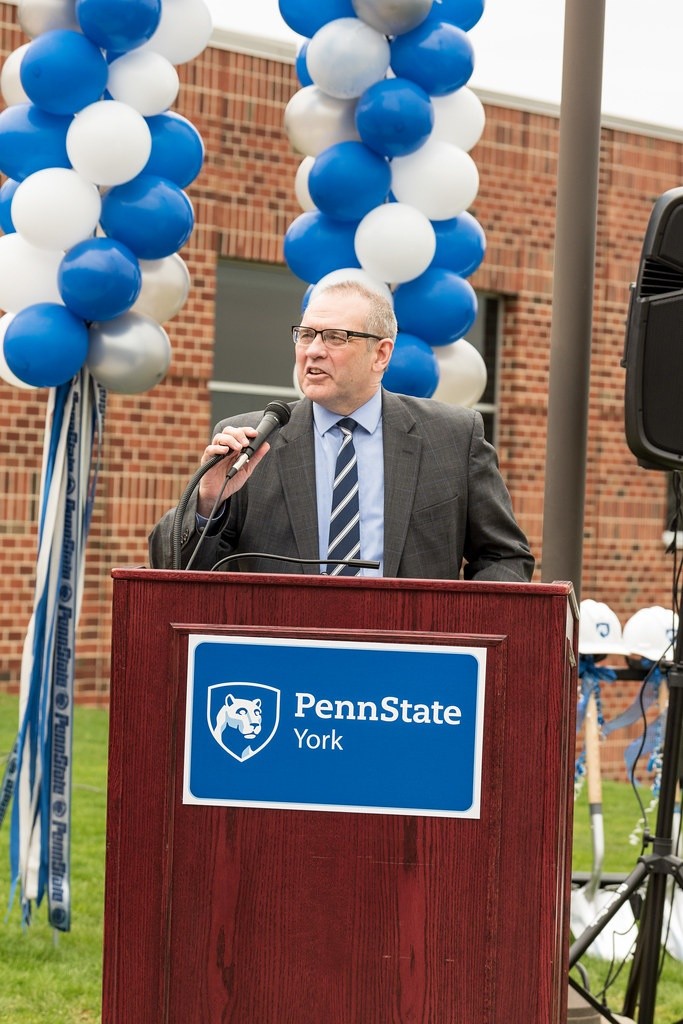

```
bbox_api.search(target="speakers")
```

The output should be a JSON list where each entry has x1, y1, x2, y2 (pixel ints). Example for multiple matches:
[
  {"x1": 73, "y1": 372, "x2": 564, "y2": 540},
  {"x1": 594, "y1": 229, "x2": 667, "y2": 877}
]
[{"x1": 620, "y1": 183, "x2": 683, "y2": 476}]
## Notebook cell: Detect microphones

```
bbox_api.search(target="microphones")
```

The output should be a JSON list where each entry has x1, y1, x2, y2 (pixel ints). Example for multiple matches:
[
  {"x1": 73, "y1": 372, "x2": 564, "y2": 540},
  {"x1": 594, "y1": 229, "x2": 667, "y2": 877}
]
[
  {"x1": 210, "y1": 553, "x2": 380, "y2": 572},
  {"x1": 227, "y1": 399, "x2": 291, "y2": 480}
]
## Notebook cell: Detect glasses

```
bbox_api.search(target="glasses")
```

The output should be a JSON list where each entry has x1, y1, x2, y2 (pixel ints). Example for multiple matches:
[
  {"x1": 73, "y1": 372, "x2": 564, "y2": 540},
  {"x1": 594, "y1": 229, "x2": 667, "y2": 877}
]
[{"x1": 291, "y1": 326, "x2": 383, "y2": 349}]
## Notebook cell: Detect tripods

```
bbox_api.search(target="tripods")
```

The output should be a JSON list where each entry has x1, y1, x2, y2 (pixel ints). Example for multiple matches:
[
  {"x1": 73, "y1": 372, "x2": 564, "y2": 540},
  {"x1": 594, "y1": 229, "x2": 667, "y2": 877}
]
[{"x1": 570, "y1": 587, "x2": 683, "y2": 1024}]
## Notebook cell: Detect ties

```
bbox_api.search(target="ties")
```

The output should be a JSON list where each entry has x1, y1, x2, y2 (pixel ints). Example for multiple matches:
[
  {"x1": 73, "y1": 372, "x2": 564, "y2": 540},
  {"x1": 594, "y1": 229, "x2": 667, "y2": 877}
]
[{"x1": 326, "y1": 417, "x2": 361, "y2": 577}]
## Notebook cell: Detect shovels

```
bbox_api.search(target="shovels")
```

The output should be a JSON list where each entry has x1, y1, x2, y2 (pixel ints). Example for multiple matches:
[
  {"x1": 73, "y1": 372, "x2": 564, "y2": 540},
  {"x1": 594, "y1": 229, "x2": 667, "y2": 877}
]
[
  {"x1": 572, "y1": 679, "x2": 640, "y2": 965},
  {"x1": 659, "y1": 662, "x2": 683, "y2": 963}
]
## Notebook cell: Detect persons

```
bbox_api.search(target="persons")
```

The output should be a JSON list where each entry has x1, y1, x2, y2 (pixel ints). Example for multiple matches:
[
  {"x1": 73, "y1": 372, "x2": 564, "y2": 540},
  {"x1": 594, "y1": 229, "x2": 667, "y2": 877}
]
[{"x1": 147, "y1": 281, "x2": 536, "y2": 584}]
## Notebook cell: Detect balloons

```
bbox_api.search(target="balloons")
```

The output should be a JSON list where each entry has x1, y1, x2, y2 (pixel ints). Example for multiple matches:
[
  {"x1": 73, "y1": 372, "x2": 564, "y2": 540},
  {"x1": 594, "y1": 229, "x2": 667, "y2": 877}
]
[
  {"x1": 0, "y1": 0, "x2": 214, "y2": 393},
  {"x1": 277, "y1": 0, "x2": 487, "y2": 412}
]
